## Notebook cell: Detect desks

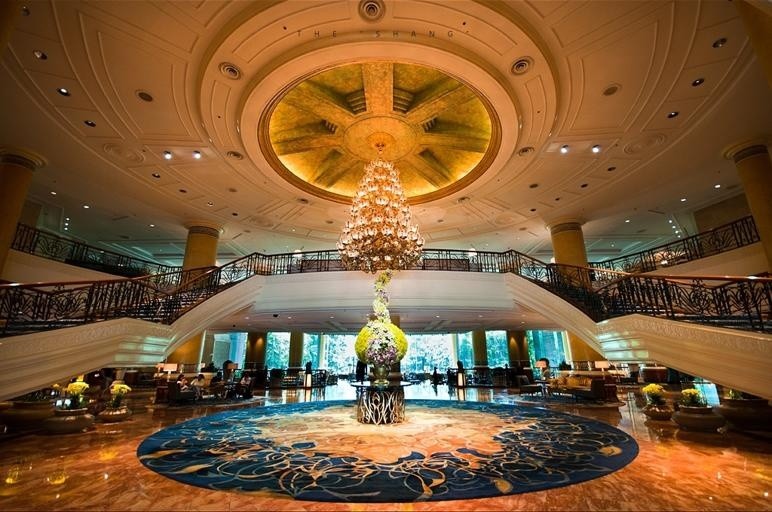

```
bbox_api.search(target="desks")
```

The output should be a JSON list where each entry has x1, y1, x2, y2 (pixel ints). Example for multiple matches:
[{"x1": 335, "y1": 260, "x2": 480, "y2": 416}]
[{"x1": 350, "y1": 378, "x2": 410, "y2": 425}]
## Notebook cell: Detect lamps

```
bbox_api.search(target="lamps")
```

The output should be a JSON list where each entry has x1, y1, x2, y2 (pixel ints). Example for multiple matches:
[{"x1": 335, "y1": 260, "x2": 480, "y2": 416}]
[
  {"x1": 227, "y1": 363, "x2": 237, "y2": 372},
  {"x1": 153, "y1": 362, "x2": 176, "y2": 378},
  {"x1": 336, "y1": 149, "x2": 425, "y2": 273}
]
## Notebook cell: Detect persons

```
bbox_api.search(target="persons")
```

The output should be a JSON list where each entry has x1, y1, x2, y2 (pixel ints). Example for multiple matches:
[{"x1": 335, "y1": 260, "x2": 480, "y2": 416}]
[{"x1": 175, "y1": 370, "x2": 253, "y2": 402}]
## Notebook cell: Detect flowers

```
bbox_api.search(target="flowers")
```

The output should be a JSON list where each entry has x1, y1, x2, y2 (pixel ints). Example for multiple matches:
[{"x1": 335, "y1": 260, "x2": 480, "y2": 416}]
[
  {"x1": 356, "y1": 270, "x2": 410, "y2": 365},
  {"x1": 19, "y1": 380, "x2": 131, "y2": 409},
  {"x1": 642, "y1": 381, "x2": 758, "y2": 405}
]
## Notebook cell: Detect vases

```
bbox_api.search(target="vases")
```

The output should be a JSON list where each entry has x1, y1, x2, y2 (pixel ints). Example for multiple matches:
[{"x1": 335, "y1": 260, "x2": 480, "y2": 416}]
[
  {"x1": 0, "y1": 402, "x2": 131, "y2": 434},
  {"x1": 640, "y1": 402, "x2": 770, "y2": 432},
  {"x1": 369, "y1": 361, "x2": 392, "y2": 384}
]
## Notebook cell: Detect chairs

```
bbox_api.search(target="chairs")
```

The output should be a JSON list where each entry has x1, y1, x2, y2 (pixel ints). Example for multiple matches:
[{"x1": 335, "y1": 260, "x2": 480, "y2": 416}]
[
  {"x1": 516, "y1": 361, "x2": 669, "y2": 404},
  {"x1": 167, "y1": 375, "x2": 257, "y2": 407}
]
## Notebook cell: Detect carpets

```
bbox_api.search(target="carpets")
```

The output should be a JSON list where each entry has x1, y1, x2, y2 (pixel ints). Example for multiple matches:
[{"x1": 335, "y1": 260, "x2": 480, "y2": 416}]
[{"x1": 135, "y1": 395, "x2": 637, "y2": 506}]
